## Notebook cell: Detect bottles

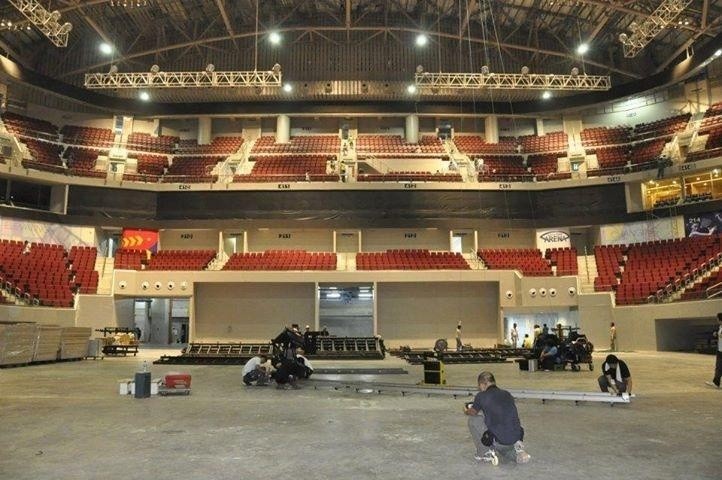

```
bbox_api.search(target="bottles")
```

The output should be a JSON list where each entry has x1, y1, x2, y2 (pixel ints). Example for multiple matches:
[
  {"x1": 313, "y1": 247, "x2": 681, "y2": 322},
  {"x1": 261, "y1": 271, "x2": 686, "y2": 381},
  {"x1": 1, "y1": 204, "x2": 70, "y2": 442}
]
[{"x1": 142, "y1": 359, "x2": 147, "y2": 372}]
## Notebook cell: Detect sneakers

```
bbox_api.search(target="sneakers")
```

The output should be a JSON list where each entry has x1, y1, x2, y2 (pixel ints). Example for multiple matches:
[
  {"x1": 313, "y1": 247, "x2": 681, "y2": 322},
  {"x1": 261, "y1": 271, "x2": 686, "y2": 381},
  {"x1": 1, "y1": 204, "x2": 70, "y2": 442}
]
[
  {"x1": 474, "y1": 449, "x2": 498, "y2": 463},
  {"x1": 513, "y1": 440, "x2": 531, "y2": 465}
]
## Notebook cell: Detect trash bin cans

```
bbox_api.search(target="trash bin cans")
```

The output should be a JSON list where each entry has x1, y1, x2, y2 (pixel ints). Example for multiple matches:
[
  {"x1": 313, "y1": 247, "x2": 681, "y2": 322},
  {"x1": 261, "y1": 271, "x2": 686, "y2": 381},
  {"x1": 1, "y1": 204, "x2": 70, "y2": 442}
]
[
  {"x1": 528, "y1": 359, "x2": 538, "y2": 372},
  {"x1": 425, "y1": 361, "x2": 440, "y2": 384},
  {"x1": 135, "y1": 371, "x2": 151, "y2": 398}
]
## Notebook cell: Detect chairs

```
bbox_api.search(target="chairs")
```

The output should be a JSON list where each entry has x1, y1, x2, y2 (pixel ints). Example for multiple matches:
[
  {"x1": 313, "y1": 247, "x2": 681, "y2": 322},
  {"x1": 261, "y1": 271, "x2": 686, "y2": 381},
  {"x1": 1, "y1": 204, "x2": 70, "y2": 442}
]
[
  {"x1": 592, "y1": 236, "x2": 722, "y2": 303},
  {"x1": 0, "y1": 237, "x2": 98, "y2": 310},
  {"x1": 356, "y1": 235, "x2": 578, "y2": 277},
  {"x1": 452, "y1": 132, "x2": 573, "y2": 182},
  {"x1": 230, "y1": 135, "x2": 345, "y2": 181},
  {"x1": 355, "y1": 135, "x2": 448, "y2": 158},
  {"x1": 218, "y1": 248, "x2": 337, "y2": 273},
  {"x1": 577, "y1": 112, "x2": 693, "y2": 168},
  {"x1": 686, "y1": 102, "x2": 722, "y2": 162},
  {"x1": 113, "y1": 248, "x2": 216, "y2": 272},
  {"x1": 2, "y1": 106, "x2": 245, "y2": 186},
  {"x1": 358, "y1": 170, "x2": 463, "y2": 182}
]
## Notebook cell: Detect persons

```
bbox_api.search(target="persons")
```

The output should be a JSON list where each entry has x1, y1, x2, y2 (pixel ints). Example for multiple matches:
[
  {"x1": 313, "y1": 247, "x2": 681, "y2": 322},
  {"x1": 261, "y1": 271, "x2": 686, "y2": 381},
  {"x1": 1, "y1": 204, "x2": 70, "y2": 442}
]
[
  {"x1": 172, "y1": 326, "x2": 178, "y2": 344},
  {"x1": 704, "y1": 313, "x2": 722, "y2": 388},
  {"x1": 240, "y1": 322, "x2": 325, "y2": 389},
  {"x1": 462, "y1": 371, "x2": 531, "y2": 466},
  {"x1": 688, "y1": 218, "x2": 718, "y2": 237},
  {"x1": 455, "y1": 320, "x2": 462, "y2": 351},
  {"x1": 597, "y1": 355, "x2": 632, "y2": 397},
  {"x1": 655, "y1": 158, "x2": 664, "y2": 179},
  {"x1": 511, "y1": 322, "x2": 520, "y2": 348},
  {"x1": 523, "y1": 323, "x2": 593, "y2": 373},
  {"x1": 610, "y1": 321, "x2": 617, "y2": 351}
]
